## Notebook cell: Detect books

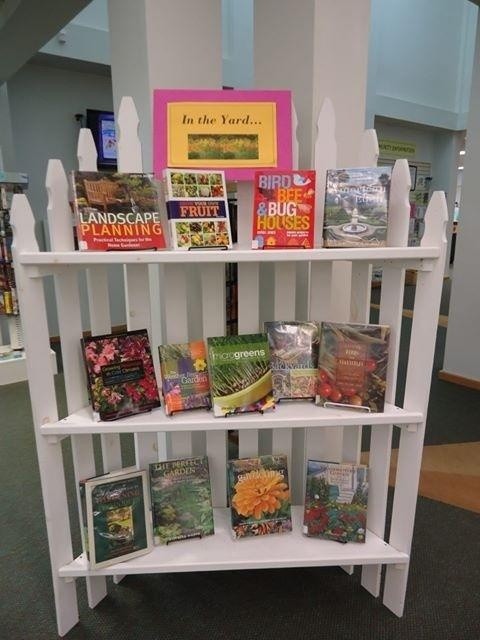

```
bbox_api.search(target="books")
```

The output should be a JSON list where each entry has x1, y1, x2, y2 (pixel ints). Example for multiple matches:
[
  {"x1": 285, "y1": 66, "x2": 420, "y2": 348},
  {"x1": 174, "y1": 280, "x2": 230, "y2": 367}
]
[
  {"x1": 70, "y1": 168, "x2": 167, "y2": 252},
  {"x1": 302, "y1": 459, "x2": 369, "y2": 544},
  {"x1": 315, "y1": 321, "x2": 390, "y2": 413},
  {"x1": 322, "y1": 166, "x2": 392, "y2": 247},
  {"x1": 79, "y1": 465, "x2": 153, "y2": 570},
  {"x1": 150, "y1": 455, "x2": 214, "y2": 545},
  {"x1": 205, "y1": 334, "x2": 274, "y2": 417},
  {"x1": 164, "y1": 169, "x2": 233, "y2": 251},
  {"x1": 264, "y1": 321, "x2": 322, "y2": 403},
  {"x1": 80, "y1": 328, "x2": 160, "y2": 421},
  {"x1": 253, "y1": 170, "x2": 316, "y2": 250},
  {"x1": 225, "y1": 454, "x2": 292, "y2": 539},
  {"x1": 157, "y1": 344, "x2": 211, "y2": 415}
]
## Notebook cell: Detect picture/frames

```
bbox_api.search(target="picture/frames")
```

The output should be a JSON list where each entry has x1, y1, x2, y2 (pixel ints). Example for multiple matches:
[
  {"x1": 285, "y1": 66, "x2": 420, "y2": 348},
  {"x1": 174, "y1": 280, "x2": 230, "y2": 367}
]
[{"x1": 153, "y1": 89, "x2": 293, "y2": 180}]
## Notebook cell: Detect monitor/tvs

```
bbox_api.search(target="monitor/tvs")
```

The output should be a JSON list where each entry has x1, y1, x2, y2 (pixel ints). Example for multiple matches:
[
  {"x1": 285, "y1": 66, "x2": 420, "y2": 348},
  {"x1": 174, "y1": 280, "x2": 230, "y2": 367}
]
[{"x1": 86, "y1": 109, "x2": 117, "y2": 169}]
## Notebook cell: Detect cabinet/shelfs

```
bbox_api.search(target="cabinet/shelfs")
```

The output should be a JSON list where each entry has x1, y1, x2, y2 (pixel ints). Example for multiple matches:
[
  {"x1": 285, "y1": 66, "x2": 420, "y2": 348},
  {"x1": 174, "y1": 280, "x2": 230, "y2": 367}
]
[{"x1": 10, "y1": 86, "x2": 449, "y2": 640}]
